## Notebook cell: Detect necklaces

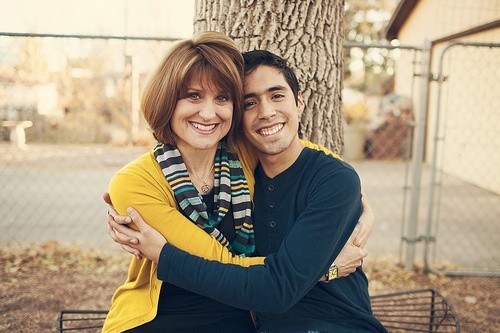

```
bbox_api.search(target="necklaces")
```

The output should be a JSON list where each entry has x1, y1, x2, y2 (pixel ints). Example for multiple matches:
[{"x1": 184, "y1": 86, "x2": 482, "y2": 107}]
[{"x1": 173, "y1": 143, "x2": 219, "y2": 193}]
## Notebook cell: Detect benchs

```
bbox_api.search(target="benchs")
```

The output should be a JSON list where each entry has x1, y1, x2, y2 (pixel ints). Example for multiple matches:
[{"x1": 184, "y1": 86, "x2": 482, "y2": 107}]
[{"x1": 58, "y1": 287, "x2": 461, "y2": 333}]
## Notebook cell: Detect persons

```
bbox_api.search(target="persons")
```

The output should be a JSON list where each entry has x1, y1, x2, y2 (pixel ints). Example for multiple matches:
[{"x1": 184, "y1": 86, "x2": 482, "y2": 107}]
[
  {"x1": 102, "y1": 49, "x2": 389, "y2": 333},
  {"x1": 102, "y1": 32, "x2": 375, "y2": 332}
]
